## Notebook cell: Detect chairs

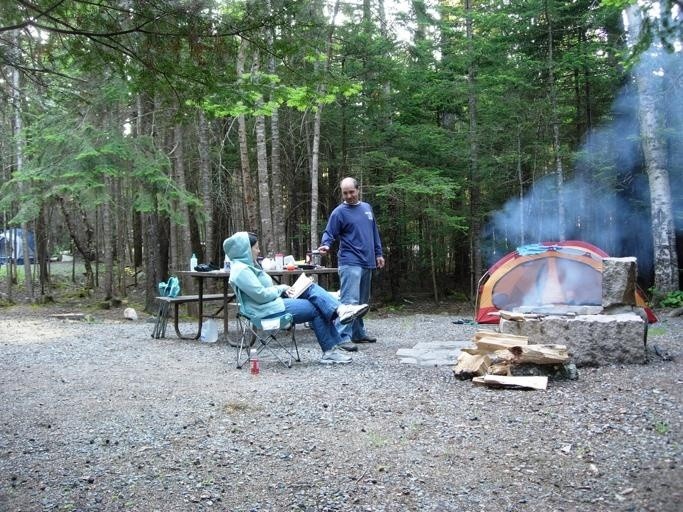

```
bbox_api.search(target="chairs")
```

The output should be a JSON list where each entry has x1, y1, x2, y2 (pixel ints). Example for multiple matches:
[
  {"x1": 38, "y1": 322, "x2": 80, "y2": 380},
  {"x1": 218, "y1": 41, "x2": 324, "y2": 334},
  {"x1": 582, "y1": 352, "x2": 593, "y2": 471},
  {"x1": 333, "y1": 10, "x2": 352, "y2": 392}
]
[{"x1": 226, "y1": 282, "x2": 302, "y2": 370}]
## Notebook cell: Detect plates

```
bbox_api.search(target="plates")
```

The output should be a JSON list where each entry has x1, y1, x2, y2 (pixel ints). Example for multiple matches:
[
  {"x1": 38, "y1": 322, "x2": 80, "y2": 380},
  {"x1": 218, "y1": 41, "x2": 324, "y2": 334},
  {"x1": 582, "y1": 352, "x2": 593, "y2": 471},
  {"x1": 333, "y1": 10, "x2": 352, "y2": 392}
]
[{"x1": 299, "y1": 265, "x2": 316, "y2": 270}]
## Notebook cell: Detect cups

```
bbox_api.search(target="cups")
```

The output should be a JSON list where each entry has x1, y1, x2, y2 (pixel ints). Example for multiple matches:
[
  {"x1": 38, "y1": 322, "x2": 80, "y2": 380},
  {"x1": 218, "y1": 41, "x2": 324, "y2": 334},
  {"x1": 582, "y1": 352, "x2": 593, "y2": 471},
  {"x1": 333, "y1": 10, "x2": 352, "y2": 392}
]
[{"x1": 305, "y1": 249, "x2": 320, "y2": 266}]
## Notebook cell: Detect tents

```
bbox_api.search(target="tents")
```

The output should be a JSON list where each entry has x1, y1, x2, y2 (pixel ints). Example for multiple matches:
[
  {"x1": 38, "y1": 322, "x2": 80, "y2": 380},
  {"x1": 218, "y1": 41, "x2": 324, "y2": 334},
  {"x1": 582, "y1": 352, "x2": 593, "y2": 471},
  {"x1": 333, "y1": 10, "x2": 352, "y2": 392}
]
[
  {"x1": 475, "y1": 240, "x2": 658, "y2": 323},
  {"x1": 0, "y1": 227, "x2": 35, "y2": 264}
]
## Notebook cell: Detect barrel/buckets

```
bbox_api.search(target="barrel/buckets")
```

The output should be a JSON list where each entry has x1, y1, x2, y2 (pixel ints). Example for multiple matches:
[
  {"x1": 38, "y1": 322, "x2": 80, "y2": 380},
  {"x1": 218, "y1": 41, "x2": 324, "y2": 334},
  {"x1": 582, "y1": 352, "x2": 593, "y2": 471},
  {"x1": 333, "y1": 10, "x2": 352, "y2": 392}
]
[{"x1": 199, "y1": 316, "x2": 219, "y2": 343}]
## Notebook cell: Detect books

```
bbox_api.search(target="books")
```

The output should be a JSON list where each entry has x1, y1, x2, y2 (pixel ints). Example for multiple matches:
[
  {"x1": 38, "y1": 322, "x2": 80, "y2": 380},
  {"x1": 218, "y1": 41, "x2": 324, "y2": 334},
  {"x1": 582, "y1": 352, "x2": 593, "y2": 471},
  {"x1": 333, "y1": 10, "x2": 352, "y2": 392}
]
[{"x1": 289, "y1": 272, "x2": 312, "y2": 299}]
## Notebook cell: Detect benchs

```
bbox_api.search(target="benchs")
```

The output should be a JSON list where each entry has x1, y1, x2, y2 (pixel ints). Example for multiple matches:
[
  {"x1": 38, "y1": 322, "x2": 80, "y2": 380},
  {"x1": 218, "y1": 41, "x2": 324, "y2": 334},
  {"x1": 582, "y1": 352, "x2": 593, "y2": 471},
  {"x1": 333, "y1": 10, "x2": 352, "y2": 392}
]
[{"x1": 150, "y1": 292, "x2": 256, "y2": 343}]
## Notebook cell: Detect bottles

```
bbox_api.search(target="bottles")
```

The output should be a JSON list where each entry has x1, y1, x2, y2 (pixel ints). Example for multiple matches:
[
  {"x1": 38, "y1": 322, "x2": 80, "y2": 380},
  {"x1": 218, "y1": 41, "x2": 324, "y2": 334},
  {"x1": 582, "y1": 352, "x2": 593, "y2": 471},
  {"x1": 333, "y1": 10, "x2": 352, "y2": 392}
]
[
  {"x1": 189, "y1": 253, "x2": 197, "y2": 272},
  {"x1": 274, "y1": 254, "x2": 284, "y2": 271},
  {"x1": 249, "y1": 349, "x2": 259, "y2": 374},
  {"x1": 223, "y1": 254, "x2": 230, "y2": 272}
]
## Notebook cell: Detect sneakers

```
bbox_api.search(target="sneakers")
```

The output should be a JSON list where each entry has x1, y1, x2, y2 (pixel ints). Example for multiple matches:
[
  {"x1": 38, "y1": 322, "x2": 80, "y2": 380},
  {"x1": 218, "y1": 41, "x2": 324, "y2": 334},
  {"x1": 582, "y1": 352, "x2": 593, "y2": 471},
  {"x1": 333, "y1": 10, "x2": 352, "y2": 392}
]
[
  {"x1": 320, "y1": 336, "x2": 376, "y2": 363},
  {"x1": 339, "y1": 304, "x2": 369, "y2": 325}
]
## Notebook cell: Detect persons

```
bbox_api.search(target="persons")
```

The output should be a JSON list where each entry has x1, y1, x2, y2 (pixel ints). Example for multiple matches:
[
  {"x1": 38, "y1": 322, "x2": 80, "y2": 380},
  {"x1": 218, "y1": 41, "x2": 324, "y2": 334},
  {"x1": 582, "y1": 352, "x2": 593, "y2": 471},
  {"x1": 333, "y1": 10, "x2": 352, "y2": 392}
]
[
  {"x1": 318, "y1": 177, "x2": 385, "y2": 351},
  {"x1": 223, "y1": 231, "x2": 369, "y2": 364}
]
